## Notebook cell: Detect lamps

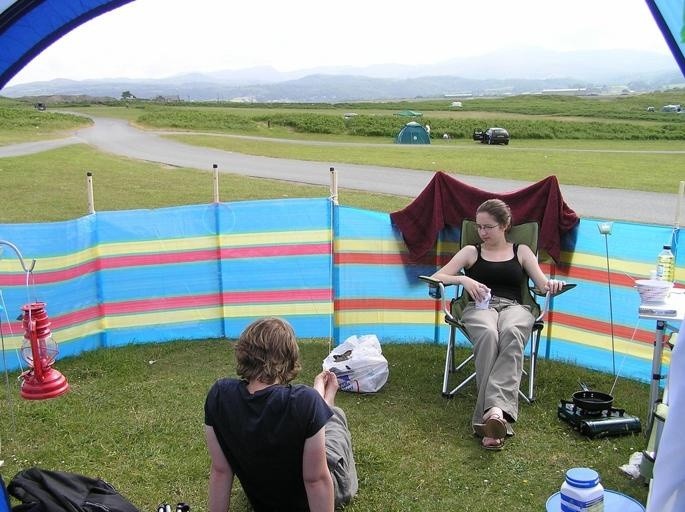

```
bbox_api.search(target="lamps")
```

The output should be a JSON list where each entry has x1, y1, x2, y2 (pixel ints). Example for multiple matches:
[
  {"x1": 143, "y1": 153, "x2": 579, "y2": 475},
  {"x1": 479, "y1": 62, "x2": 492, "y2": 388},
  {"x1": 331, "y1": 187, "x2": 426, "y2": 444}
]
[{"x1": 17, "y1": 267, "x2": 69, "y2": 400}]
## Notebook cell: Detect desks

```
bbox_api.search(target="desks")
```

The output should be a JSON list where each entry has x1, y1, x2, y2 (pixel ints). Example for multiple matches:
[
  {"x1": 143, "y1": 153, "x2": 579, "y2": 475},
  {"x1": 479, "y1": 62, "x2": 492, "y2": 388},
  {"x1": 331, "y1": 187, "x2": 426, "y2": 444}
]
[{"x1": 639, "y1": 288, "x2": 685, "y2": 440}]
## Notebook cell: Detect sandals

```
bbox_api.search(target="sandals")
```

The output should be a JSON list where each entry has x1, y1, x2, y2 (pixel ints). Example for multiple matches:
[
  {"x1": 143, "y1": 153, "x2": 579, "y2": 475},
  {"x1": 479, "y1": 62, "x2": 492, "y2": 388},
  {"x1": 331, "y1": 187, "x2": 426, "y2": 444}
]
[{"x1": 481, "y1": 414, "x2": 506, "y2": 450}]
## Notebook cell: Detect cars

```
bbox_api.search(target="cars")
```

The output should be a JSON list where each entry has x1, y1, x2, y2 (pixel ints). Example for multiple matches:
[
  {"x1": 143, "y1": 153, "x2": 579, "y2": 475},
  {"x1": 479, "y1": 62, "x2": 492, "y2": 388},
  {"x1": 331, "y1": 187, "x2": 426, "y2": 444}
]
[{"x1": 473, "y1": 127, "x2": 509, "y2": 145}]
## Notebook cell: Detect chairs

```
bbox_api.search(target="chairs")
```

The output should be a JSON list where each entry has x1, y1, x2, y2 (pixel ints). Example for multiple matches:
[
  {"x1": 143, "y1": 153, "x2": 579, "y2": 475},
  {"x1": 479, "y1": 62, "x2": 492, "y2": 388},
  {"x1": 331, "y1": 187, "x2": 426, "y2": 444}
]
[{"x1": 417, "y1": 219, "x2": 578, "y2": 406}]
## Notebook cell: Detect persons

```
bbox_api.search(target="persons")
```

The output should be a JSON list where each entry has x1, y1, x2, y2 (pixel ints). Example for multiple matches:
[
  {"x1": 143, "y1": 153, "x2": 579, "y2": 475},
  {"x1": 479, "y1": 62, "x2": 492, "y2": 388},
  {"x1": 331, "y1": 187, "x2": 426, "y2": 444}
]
[
  {"x1": 200, "y1": 314, "x2": 359, "y2": 511},
  {"x1": 428, "y1": 197, "x2": 568, "y2": 450}
]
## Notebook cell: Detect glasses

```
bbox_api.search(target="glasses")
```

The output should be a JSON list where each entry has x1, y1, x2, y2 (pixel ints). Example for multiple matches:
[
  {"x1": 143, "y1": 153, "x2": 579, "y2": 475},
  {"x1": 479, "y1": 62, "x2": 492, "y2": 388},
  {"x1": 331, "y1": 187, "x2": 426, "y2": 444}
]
[{"x1": 474, "y1": 222, "x2": 502, "y2": 231}]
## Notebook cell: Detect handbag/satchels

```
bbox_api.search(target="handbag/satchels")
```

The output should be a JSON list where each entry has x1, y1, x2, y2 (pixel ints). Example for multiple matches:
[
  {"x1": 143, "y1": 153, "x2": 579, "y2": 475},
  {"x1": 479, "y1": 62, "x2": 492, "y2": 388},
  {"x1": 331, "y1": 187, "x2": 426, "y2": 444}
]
[{"x1": 6, "y1": 468, "x2": 138, "y2": 512}]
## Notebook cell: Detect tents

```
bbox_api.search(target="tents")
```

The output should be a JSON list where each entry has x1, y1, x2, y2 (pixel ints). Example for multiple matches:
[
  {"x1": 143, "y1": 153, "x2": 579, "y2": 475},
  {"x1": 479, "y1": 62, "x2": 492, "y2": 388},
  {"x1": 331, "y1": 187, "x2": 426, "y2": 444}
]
[{"x1": 395, "y1": 121, "x2": 431, "y2": 145}]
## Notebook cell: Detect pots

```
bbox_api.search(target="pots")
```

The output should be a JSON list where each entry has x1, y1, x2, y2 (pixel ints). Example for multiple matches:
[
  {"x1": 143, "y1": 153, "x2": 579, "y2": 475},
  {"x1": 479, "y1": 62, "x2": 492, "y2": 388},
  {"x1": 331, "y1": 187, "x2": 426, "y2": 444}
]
[{"x1": 572, "y1": 381, "x2": 614, "y2": 412}]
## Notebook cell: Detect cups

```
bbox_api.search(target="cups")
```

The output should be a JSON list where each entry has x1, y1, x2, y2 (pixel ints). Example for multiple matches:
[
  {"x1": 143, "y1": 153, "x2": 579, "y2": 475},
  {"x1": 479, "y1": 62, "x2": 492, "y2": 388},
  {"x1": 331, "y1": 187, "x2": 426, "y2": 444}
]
[{"x1": 475, "y1": 288, "x2": 492, "y2": 310}]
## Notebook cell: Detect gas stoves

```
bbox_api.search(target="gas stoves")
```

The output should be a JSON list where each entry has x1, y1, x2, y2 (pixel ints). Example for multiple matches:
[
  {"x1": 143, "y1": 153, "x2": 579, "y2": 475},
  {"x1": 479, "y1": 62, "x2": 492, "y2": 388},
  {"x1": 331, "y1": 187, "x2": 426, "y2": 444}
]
[{"x1": 557, "y1": 399, "x2": 642, "y2": 438}]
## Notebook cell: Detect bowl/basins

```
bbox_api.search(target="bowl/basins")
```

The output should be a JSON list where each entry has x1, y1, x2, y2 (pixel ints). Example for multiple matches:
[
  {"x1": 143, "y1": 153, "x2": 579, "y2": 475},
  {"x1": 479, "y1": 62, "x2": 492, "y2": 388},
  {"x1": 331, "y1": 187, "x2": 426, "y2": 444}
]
[{"x1": 634, "y1": 279, "x2": 673, "y2": 306}]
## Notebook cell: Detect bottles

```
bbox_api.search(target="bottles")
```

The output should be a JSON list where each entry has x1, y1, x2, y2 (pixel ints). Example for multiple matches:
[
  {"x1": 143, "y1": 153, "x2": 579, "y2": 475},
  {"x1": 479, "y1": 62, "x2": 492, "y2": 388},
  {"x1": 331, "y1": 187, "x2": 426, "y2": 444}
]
[
  {"x1": 560, "y1": 468, "x2": 604, "y2": 512},
  {"x1": 655, "y1": 246, "x2": 675, "y2": 283}
]
